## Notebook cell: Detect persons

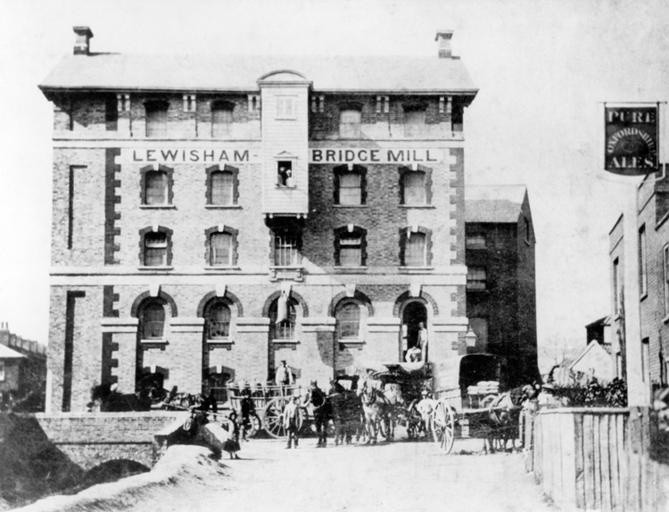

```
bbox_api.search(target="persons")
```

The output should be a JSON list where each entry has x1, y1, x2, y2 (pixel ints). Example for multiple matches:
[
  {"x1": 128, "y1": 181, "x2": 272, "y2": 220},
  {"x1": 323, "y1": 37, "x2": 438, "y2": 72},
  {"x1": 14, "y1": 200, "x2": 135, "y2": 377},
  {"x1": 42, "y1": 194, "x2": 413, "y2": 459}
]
[
  {"x1": 239, "y1": 384, "x2": 252, "y2": 396},
  {"x1": 402, "y1": 346, "x2": 423, "y2": 363},
  {"x1": 237, "y1": 388, "x2": 260, "y2": 442},
  {"x1": 197, "y1": 388, "x2": 220, "y2": 424},
  {"x1": 414, "y1": 321, "x2": 430, "y2": 363},
  {"x1": 251, "y1": 383, "x2": 265, "y2": 398},
  {"x1": 223, "y1": 410, "x2": 243, "y2": 461},
  {"x1": 262, "y1": 383, "x2": 279, "y2": 401},
  {"x1": 273, "y1": 359, "x2": 298, "y2": 396},
  {"x1": 279, "y1": 396, "x2": 304, "y2": 451}
]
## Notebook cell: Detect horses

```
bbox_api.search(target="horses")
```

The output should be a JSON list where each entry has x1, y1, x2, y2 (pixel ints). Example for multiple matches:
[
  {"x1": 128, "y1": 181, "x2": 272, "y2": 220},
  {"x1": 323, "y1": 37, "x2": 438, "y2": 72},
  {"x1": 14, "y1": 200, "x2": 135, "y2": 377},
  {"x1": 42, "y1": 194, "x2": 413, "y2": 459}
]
[
  {"x1": 88, "y1": 380, "x2": 141, "y2": 410},
  {"x1": 150, "y1": 383, "x2": 218, "y2": 421},
  {"x1": 307, "y1": 376, "x2": 397, "y2": 448},
  {"x1": 480, "y1": 382, "x2": 542, "y2": 453}
]
